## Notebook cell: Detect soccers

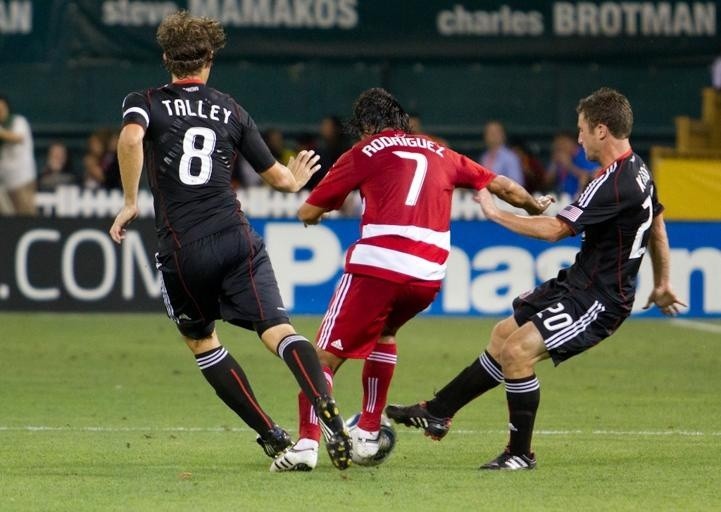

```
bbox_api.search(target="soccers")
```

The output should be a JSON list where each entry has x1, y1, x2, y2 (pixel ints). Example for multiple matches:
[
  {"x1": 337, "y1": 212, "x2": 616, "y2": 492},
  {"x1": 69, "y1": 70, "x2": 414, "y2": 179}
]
[{"x1": 344, "y1": 412, "x2": 396, "y2": 466}]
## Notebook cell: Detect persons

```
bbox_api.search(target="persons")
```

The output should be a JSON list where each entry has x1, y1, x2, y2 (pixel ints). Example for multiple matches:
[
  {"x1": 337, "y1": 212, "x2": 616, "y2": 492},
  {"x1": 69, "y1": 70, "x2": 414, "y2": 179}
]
[
  {"x1": 109, "y1": 10, "x2": 353, "y2": 470},
  {"x1": 269, "y1": 87, "x2": 556, "y2": 473},
  {"x1": 2, "y1": 100, "x2": 600, "y2": 216},
  {"x1": 384, "y1": 86, "x2": 688, "y2": 470}
]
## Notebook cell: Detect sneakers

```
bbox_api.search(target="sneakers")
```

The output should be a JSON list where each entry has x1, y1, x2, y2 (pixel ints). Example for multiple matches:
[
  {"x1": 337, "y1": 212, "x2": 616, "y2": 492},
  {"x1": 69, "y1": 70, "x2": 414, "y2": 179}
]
[
  {"x1": 481, "y1": 445, "x2": 536, "y2": 471},
  {"x1": 386, "y1": 402, "x2": 452, "y2": 442},
  {"x1": 256, "y1": 398, "x2": 381, "y2": 474}
]
